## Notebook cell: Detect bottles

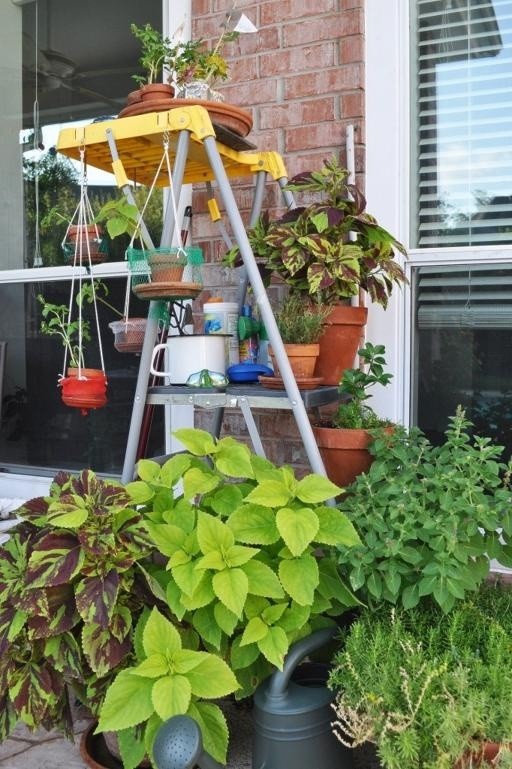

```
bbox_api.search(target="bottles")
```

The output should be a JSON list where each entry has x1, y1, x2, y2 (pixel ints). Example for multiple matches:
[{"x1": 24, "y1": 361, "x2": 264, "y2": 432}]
[{"x1": 202, "y1": 302, "x2": 240, "y2": 366}]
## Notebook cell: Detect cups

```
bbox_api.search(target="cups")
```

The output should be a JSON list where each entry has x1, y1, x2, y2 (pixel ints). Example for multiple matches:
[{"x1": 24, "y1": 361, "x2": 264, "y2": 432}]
[{"x1": 149, "y1": 333, "x2": 234, "y2": 387}]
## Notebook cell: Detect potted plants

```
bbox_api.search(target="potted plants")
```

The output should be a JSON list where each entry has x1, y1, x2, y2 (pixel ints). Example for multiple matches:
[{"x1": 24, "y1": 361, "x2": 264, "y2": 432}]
[
  {"x1": 49, "y1": 210, "x2": 104, "y2": 255},
  {"x1": 311, "y1": 342, "x2": 398, "y2": 493},
  {"x1": 165, "y1": 29, "x2": 226, "y2": 102},
  {"x1": 0, "y1": 470, "x2": 169, "y2": 769},
  {"x1": 255, "y1": 294, "x2": 338, "y2": 378},
  {"x1": 76, "y1": 279, "x2": 150, "y2": 352},
  {"x1": 220, "y1": 151, "x2": 412, "y2": 388},
  {"x1": 130, "y1": 21, "x2": 176, "y2": 99},
  {"x1": 38, "y1": 296, "x2": 110, "y2": 410}
]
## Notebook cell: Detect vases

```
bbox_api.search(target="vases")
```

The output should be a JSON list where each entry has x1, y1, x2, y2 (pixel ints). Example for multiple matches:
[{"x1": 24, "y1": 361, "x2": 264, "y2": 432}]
[
  {"x1": 148, "y1": 255, "x2": 186, "y2": 283},
  {"x1": 128, "y1": 90, "x2": 141, "y2": 104}
]
